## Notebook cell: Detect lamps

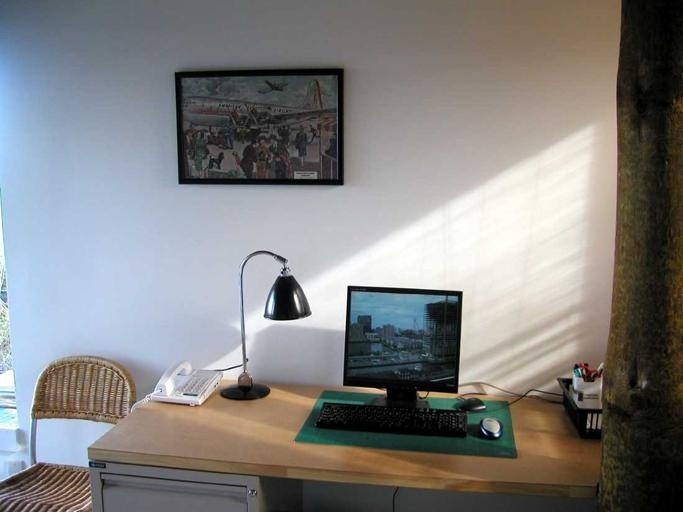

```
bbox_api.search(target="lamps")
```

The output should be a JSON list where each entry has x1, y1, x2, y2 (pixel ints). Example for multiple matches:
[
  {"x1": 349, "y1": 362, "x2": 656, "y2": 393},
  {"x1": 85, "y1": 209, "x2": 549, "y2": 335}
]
[{"x1": 220, "y1": 250, "x2": 312, "y2": 400}]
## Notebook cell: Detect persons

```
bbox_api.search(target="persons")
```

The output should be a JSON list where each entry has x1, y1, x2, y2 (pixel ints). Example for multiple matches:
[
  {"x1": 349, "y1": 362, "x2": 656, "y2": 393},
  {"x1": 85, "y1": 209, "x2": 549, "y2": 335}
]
[{"x1": 185, "y1": 108, "x2": 320, "y2": 179}]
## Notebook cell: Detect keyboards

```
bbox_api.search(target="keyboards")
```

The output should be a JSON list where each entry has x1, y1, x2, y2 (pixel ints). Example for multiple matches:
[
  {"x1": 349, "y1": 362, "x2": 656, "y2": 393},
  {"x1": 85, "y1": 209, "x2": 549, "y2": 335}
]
[{"x1": 314, "y1": 402, "x2": 468, "y2": 437}]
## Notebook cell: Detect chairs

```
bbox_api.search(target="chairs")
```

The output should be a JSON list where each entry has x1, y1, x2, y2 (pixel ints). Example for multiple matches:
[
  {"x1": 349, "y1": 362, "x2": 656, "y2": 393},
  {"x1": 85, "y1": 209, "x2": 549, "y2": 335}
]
[{"x1": 0, "y1": 355, "x2": 137, "y2": 511}]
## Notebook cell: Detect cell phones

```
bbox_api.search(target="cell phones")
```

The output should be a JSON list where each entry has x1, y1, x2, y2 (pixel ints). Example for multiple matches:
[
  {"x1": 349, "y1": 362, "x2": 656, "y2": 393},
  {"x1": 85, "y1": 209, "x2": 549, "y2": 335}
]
[{"x1": 458, "y1": 395, "x2": 485, "y2": 409}]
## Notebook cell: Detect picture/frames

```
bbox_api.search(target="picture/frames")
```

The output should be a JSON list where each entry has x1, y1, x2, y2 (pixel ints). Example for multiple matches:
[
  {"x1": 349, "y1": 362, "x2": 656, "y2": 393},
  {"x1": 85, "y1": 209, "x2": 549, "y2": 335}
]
[{"x1": 174, "y1": 68, "x2": 344, "y2": 186}]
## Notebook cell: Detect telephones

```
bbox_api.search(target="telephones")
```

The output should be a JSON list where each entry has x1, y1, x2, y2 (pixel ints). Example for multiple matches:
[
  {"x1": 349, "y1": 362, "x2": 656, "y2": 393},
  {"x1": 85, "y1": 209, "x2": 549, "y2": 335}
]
[{"x1": 150, "y1": 361, "x2": 223, "y2": 406}]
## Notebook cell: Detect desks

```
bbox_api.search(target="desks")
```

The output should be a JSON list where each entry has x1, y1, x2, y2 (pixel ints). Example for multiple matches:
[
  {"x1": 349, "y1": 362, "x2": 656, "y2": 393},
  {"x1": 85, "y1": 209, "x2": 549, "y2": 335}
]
[{"x1": 87, "y1": 379, "x2": 603, "y2": 511}]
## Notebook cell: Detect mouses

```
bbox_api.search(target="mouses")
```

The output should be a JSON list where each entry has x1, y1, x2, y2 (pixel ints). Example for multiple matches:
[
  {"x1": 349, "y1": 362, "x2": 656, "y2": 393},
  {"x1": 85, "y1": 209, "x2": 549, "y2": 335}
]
[{"x1": 481, "y1": 417, "x2": 504, "y2": 439}]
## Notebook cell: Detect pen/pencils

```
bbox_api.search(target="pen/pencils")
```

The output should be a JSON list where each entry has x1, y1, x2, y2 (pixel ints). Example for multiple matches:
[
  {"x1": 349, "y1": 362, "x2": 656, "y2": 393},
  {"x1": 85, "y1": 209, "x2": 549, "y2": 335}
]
[{"x1": 573, "y1": 362, "x2": 603, "y2": 382}]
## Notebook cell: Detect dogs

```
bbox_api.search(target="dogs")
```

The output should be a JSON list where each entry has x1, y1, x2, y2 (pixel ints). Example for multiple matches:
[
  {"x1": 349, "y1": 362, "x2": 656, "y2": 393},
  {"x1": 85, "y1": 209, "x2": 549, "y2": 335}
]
[{"x1": 208, "y1": 151, "x2": 224, "y2": 169}]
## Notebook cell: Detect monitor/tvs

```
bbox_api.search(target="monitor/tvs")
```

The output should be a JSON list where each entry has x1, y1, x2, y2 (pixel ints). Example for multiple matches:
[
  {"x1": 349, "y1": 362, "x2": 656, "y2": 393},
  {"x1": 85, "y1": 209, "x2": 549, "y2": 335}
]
[{"x1": 342, "y1": 285, "x2": 463, "y2": 407}]
